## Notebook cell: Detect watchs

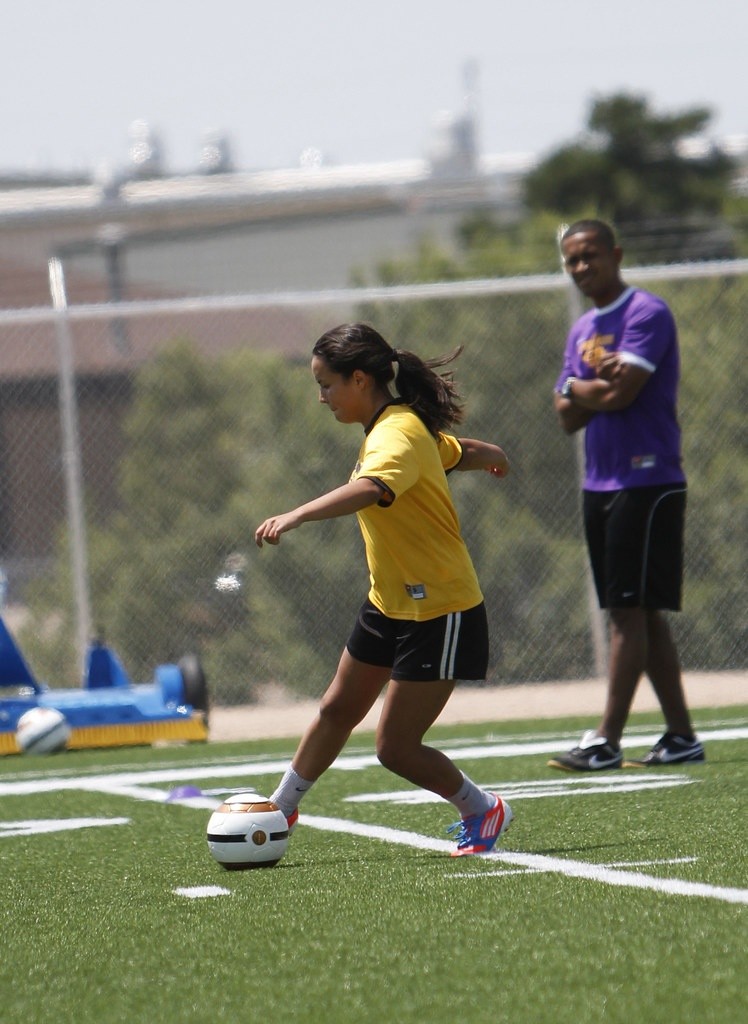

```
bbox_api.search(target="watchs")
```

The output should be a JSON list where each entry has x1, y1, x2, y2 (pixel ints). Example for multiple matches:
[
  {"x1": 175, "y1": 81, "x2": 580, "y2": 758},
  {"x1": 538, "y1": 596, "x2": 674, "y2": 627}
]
[{"x1": 562, "y1": 380, "x2": 574, "y2": 397}]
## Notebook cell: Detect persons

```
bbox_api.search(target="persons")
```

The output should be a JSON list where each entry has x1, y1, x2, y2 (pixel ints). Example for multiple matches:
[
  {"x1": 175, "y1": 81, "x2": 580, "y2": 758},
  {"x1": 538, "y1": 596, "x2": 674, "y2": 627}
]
[
  {"x1": 546, "y1": 219, "x2": 705, "y2": 771},
  {"x1": 253, "y1": 322, "x2": 513, "y2": 856}
]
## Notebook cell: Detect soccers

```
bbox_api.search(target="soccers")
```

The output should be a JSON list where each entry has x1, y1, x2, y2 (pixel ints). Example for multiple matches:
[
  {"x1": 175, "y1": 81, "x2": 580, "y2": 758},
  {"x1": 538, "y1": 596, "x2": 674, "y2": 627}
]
[
  {"x1": 16, "y1": 706, "x2": 70, "y2": 758},
  {"x1": 207, "y1": 793, "x2": 290, "y2": 871}
]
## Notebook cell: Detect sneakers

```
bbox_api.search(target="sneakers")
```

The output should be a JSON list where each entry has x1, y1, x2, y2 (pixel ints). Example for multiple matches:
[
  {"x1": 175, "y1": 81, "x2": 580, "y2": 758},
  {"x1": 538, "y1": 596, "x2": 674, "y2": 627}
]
[
  {"x1": 286, "y1": 808, "x2": 298, "y2": 837},
  {"x1": 446, "y1": 792, "x2": 514, "y2": 858},
  {"x1": 622, "y1": 731, "x2": 705, "y2": 769},
  {"x1": 547, "y1": 730, "x2": 624, "y2": 773}
]
